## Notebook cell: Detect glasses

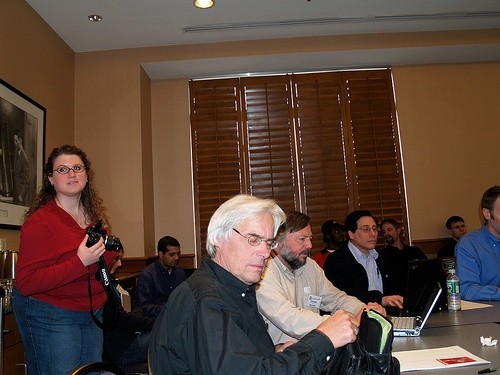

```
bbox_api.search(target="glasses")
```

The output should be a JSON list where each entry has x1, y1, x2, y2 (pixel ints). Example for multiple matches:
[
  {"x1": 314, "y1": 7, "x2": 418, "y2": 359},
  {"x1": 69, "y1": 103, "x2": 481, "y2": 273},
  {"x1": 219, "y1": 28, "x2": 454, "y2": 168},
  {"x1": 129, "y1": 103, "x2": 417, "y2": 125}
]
[
  {"x1": 51, "y1": 163, "x2": 87, "y2": 173},
  {"x1": 233, "y1": 228, "x2": 279, "y2": 250}
]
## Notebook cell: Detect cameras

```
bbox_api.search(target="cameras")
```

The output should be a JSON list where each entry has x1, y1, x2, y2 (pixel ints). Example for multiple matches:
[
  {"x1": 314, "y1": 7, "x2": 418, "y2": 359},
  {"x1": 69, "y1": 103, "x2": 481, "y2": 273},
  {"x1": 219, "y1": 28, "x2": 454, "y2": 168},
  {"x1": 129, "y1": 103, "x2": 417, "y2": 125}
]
[{"x1": 87, "y1": 219, "x2": 121, "y2": 253}]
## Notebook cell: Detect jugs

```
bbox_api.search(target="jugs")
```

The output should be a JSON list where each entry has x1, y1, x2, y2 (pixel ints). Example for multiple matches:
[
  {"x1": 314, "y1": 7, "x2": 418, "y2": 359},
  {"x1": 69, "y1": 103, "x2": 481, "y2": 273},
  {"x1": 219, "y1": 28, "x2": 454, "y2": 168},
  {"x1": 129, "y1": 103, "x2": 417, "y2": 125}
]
[{"x1": 3, "y1": 250, "x2": 19, "y2": 280}]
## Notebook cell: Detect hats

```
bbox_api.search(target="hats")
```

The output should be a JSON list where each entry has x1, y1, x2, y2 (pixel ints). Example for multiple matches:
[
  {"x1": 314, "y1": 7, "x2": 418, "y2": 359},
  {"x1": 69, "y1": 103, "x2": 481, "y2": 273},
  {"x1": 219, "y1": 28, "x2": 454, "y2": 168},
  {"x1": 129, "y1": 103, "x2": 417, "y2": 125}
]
[{"x1": 321, "y1": 220, "x2": 345, "y2": 242}]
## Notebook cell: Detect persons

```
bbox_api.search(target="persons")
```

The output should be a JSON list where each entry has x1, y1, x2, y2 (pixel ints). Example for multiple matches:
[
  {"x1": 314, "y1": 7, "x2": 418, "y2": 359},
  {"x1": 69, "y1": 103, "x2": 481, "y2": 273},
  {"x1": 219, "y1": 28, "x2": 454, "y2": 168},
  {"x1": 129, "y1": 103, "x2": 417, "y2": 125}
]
[
  {"x1": 319, "y1": 209, "x2": 404, "y2": 316},
  {"x1": 103, "y1": 237, "x2": 149, "y2": 375},
  {"x1": 437, "y1": 216, "x2": 468, "y2": 260},
  {"x1": 10, "y1": 145, "x2": 120, "y2": 375},
  {"x1": 377, "y1": 216, "x2": 430, "y2": 283},
  {"x1": 137, "y1": 235, "x2": 185, "y2": 308},
  {"x1": 257, "y1": 209, "x2": 388, "y2": 352},
  {"x1": 145, "y1": 194, "x2": 360, "y2": 374},
  {"x1": 454, "y1": 185, "x2": 499, "y2": 301},
  {"x1": 11, "y1": 132, "x2": 34, "y2": 204}
]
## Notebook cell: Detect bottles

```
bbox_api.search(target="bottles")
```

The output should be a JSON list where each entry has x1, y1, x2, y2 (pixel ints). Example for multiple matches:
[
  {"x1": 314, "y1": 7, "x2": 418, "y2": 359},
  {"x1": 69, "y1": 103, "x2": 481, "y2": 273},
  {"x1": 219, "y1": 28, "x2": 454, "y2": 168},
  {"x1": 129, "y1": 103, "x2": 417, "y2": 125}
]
[
  {"x1": 2, "y1": 281, "x2": 14, "y2": 311},
  {"x1": 446, "y1": 269, "x2": 461, "y2": 313}
]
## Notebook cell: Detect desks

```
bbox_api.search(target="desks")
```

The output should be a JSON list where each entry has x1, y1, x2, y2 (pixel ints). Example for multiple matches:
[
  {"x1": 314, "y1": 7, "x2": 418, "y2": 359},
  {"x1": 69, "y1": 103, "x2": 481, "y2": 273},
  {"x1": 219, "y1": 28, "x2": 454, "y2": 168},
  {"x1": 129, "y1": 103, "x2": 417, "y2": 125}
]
[{"x1": 393, "y1": 299, "x2": 500, "y2": 375}]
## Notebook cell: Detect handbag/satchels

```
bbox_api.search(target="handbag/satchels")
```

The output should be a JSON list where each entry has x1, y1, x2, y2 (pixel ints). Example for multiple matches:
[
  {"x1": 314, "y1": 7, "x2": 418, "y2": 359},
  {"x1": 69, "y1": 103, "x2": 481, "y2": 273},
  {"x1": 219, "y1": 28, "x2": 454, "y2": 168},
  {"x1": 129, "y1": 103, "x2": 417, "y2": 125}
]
[
  {"x1": 321, "y1": 335, "x2": 400, "y2": 374},
  {"x1": 360, "y1": 308, "x2": 394, "y2": 354}
]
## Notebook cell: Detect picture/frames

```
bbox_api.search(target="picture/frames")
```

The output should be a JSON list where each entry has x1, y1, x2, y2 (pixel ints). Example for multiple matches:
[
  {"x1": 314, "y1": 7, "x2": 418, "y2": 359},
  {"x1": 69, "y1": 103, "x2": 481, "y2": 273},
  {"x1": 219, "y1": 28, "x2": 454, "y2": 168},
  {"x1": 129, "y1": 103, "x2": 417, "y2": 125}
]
[{"x1": 0, "y1": 78, "x2": 47, "y2": 231}]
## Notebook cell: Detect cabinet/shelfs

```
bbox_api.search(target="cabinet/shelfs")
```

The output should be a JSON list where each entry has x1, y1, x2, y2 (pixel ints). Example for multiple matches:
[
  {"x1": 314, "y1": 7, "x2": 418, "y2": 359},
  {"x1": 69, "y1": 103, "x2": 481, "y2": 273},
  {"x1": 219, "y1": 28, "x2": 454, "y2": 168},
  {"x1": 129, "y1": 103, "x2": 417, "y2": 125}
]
[{"x1": 2, "y1": 270, "x2": 142, "y2": 374}]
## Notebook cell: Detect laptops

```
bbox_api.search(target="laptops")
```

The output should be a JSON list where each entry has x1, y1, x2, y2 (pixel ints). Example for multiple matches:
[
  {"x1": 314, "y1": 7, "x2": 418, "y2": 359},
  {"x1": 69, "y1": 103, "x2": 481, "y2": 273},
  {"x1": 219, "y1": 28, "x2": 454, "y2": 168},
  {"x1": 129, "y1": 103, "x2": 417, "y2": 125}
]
[{"x1": 391, "y1": 282, "x2": 443, "y2": 337}]
